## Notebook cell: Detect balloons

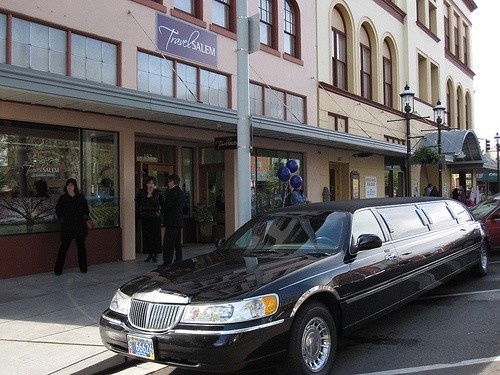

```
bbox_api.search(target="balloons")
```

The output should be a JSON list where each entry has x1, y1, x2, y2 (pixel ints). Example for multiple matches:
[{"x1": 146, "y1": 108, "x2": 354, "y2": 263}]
[
  {"x1": 291, "y1": 175, "x2": 302, "y2": 188},
  {"x1": 278, "y1": 167, "x2": 291, "y2": 182},
  {"x1": 286, "y1": 160, "x2": 298, "y2": 173}
]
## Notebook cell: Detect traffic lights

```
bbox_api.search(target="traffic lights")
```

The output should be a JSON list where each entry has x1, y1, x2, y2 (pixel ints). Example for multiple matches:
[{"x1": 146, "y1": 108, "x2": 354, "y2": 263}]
[{"x1": 485, "y1": 139, "x2": 491, "y2": 152}]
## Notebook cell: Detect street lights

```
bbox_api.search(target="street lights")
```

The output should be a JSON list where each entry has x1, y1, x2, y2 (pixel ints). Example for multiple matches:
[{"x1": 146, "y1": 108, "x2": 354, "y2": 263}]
[
  {"x1": 399, "y1": 81, "x2": 415, "y2": 197},
  {"x1": 432, "y1": 99, "x2": 446, "y2": 197},
  {"x1": 493, "y1": 131, "x2": 500, "y2": 192}
]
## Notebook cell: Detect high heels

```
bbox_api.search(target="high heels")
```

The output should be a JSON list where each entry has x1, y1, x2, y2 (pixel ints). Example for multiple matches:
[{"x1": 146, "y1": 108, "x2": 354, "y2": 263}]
[{"x1": 143, "y1": 254, "x2": 158, "y2": 263}]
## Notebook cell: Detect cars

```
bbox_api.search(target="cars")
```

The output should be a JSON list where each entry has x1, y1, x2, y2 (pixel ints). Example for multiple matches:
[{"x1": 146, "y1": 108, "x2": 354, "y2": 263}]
[
  {"x1": 469, "y1": 192, "x2": 500, "y2": 257},
  {"x1": 99, "y1": 196, "x2": 491, "y2": 375}
]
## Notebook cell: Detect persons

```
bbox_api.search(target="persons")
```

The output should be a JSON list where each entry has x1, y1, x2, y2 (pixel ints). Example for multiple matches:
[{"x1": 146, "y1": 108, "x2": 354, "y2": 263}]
[
  {"x1": 55, "y1": 178, "x2": 87, "y2": 275},
  {"x1": 34, "y1": 180, "x2": 49, "y2": 198},
  {"x1": 291, "y1": 186, "x2": 304, "y2": 204},
  {"x1": 425, "y1": 184, "x2": 432, "y2": 196},
  {"x1": 160, "y1": 174, "x2": 185, "y2": 267},
  {"x1": 430, "y1": 186, "x2": 439, "y2": 196},
  {"x1": 322, "y1": 187, "x2": 331, "y2": 202},
  {"x1": 453, "y1": 188, "x2": 460, "y2": 200},
  {"x1": 138, "y1": 176, "x2": 164, "y2": 263}
]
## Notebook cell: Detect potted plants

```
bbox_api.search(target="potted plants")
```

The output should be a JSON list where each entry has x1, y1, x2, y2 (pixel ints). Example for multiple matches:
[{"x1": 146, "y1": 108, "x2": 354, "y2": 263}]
[{"x1": 408, "y1": 145, "x2": 445, "y2": 165}]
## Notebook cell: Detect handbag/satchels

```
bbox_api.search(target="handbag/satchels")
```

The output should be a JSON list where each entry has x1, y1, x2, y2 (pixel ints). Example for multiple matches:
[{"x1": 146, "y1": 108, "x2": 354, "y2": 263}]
[{"x1": 85, "y1": 216, "x2": 93, "y2": 230}]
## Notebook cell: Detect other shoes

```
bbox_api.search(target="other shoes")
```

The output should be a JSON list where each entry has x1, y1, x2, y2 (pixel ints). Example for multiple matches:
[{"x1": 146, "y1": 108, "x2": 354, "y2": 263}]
[
  {"x1": 55, "y1": 269, "x2": 62, "y2": 275},
  {"x1": 158, "y1": 263, "x2": 171, "y2": 267},
  {"x1": 80, "y1": 267, "x2": 87, "y2": 273}
]
